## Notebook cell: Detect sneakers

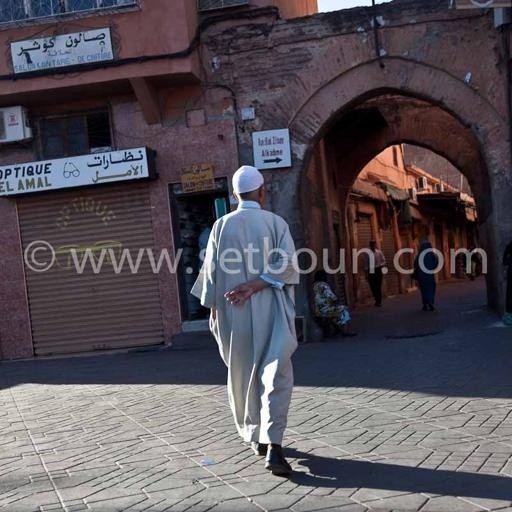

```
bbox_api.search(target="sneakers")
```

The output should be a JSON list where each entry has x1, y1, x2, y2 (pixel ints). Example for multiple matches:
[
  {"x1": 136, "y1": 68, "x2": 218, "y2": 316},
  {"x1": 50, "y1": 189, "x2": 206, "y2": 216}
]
[{"x1": 251, "y1": 441, "x2": 292, "y2": 473}]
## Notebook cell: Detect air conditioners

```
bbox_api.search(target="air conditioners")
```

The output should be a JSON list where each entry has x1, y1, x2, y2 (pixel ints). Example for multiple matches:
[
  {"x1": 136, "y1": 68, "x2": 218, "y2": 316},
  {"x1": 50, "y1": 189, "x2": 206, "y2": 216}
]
[
  {"x1": 435, "y1": 183, "x2": 444, "y2": 192},
  {"x1": 408, "y1": 187, "x2": 417, "y2": 201},
  {"x1": 418, "y1": 176, "x2": 427, "y2": 190}
]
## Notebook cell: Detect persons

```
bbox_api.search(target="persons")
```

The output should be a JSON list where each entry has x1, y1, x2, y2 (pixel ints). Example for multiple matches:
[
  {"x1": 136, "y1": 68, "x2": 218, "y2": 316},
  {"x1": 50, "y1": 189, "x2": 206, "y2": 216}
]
[
  {"x1": 413, "y1": 241, "x2": 438, "y2": 312},
  {"x1": 363, "y1": 241, "x2": 387, "y2": 307},
  {"x1": 310, "y1": 270, "x2": 357, "y2": 336},
  {"x1": 188, "y1": 164, "x2": 301, "y2": 477}
]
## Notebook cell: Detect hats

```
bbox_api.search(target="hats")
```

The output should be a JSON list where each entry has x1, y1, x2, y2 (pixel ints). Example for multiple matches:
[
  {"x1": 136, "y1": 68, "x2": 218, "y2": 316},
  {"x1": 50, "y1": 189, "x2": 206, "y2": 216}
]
[{"x1": 232, "y1": 165, "x2": 264, "y2": 194}]
